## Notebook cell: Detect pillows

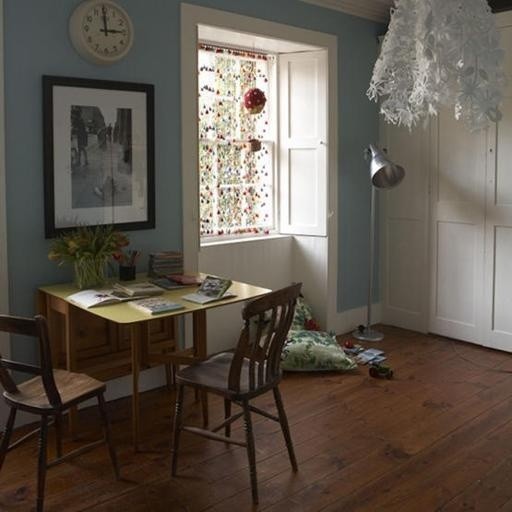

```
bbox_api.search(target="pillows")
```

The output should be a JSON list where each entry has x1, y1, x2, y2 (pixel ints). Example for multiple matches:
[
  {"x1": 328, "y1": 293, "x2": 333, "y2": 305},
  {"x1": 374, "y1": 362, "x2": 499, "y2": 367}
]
[
  {"x1": 244, "y1": 290, "x2": 321, "y2": 334},
  {"x1": 260, "y1": 328, "x2": 359, "y2": 372}
]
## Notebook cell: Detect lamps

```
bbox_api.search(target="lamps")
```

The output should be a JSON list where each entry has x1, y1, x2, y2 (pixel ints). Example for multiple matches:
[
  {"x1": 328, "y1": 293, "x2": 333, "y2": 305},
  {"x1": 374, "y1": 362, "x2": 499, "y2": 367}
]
[{"x1": 353, "y1": 144, "x2": 405, "y2": 343}]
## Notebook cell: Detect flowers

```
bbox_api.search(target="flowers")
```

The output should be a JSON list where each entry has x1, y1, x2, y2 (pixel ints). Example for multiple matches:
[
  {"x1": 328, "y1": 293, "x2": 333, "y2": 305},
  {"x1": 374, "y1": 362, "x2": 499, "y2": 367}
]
[{"x1": 46, "y1": 220, "x2": 130, "y2": 290}]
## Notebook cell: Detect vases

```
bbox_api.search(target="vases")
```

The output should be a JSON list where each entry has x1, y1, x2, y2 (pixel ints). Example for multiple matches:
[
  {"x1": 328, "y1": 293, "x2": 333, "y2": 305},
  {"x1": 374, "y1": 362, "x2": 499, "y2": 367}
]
[{"x1": 73, "y1": 259, "x2": 120, "y2": 290}]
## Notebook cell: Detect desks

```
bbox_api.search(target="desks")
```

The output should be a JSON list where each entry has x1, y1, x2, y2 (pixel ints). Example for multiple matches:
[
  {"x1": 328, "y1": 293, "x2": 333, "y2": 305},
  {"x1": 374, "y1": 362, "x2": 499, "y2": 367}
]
[{"x1": 37, "y1": 269, "x2": 273, "y2": 453}]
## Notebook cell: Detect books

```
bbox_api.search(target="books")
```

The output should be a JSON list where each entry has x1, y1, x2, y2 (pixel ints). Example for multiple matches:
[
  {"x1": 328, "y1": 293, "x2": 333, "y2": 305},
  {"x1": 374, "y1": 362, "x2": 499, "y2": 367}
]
[{"x1": 64, "y1": 250, "x2": 238, "y2": 317}]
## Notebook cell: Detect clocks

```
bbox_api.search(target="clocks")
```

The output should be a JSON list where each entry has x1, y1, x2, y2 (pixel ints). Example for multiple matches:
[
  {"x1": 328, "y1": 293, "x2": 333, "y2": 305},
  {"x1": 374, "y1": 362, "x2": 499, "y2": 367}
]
[{"x1": 68, "y1": 0, "x2": 134, "y2": 65}]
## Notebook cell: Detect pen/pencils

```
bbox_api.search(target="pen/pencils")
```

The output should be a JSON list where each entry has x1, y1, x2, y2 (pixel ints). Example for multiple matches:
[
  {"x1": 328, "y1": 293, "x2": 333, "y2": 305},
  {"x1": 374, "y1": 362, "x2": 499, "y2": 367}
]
[{"x1": 127, "y1": 249, "x2": 141, "y2": 263}]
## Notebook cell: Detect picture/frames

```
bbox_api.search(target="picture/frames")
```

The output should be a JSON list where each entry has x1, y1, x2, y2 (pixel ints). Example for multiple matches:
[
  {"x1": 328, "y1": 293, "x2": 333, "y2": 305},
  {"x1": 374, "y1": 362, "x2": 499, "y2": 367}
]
[{"x1": 43, "y1": 75, "x2": 155, "y2": 240}]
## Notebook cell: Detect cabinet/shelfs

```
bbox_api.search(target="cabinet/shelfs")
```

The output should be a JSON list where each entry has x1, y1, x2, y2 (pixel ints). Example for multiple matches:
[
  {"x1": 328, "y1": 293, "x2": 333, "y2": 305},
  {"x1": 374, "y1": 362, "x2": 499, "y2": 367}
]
[
  {"x1": 46, "y1": 294, "x2": 179, "y2": 420},
  {"x1": 379, "y1": 11, "x2": 512, "y2": 354}
]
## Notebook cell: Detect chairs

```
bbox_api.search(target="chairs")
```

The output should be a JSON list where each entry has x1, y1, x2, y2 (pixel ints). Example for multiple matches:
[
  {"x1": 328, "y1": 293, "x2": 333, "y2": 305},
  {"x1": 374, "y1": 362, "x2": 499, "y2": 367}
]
[
  {"x1": 171, "y1": 282, "x2": 303, "y2": 506},
  {"x1": 0, "y1": 313, "x2": 120, "y2": 512}
]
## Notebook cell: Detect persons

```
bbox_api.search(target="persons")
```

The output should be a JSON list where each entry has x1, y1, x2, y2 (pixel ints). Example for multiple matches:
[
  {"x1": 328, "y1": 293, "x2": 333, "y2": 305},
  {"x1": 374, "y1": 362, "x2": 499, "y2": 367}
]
[
  {"x1": 76, "y1": 117, "x2": 90, "y2": 168},
  {"x1": 107, "y1": 122, "x2": 113, "y2": 145}
]
